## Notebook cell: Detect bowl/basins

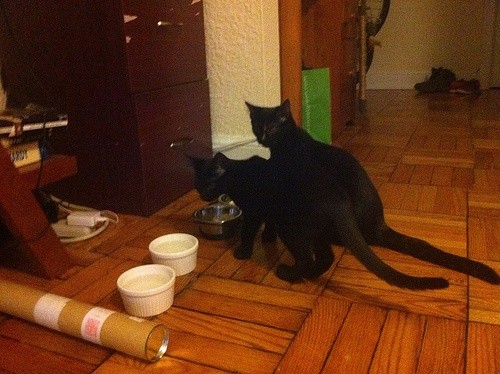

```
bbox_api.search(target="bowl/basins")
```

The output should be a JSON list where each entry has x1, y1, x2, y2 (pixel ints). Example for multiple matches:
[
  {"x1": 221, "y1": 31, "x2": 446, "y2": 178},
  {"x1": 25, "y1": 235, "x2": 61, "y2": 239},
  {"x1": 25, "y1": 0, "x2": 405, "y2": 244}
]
[
  {"x1": 117, "y1": 265, "x2": 176, "y2": 318},
  {"x1": 194, "y1": 203, "x2": 242, "y2": 241},
  {"x1": 149, "y1": 233, "x2": 199, "y2": 278}
]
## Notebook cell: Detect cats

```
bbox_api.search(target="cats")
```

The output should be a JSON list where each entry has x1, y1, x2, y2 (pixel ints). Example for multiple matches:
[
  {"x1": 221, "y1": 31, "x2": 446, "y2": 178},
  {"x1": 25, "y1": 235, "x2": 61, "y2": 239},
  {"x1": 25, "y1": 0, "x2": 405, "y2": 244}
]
[{"x1": 185, "y1": 99, "x2": 500, "y2": 292}]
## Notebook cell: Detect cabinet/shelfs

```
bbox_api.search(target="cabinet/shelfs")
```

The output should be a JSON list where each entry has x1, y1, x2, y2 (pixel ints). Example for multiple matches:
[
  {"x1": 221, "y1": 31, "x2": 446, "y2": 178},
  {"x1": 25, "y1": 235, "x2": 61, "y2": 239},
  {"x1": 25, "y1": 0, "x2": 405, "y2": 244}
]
[{"x1": 0, "y1": 0, "x2": 214, "y2": 217}]
